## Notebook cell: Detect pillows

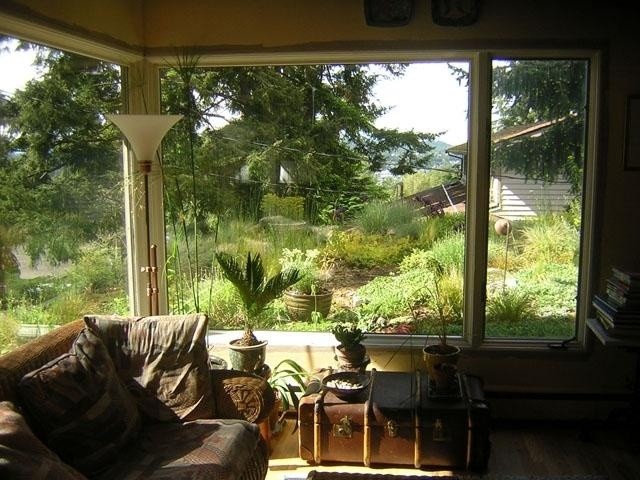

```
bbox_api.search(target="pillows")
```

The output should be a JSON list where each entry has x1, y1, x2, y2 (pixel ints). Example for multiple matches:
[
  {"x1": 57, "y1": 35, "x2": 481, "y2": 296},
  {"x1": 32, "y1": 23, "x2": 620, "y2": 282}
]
[
  {"x1": 19, "y1": 316, "x2": 142, "y2": 480},
  {"x1": 0, "y1": 400, "x2": 86, "y2": 480},
  {"x1": 84, "y1": 312, "x2": 216, "y2": 420}
]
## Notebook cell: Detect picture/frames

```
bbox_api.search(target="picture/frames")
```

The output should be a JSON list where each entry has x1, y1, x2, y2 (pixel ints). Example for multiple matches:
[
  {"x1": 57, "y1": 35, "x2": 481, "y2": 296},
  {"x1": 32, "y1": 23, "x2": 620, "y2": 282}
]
[{"x1": 623, "y1": 94, "x2": 640, "y2": 172}]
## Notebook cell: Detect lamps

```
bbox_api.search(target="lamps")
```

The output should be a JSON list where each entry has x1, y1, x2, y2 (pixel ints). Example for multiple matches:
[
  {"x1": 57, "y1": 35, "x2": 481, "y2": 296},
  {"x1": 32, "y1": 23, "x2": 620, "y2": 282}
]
[{"x1": 104, "y1": 113, "x2": 186, "y2": 317}]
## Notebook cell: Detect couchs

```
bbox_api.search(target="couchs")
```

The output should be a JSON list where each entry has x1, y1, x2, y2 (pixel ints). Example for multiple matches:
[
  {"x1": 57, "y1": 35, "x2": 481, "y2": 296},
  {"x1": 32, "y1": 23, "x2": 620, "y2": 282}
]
[{"x1": 1, "y1": 317, "x2": 274, "y2": 479}]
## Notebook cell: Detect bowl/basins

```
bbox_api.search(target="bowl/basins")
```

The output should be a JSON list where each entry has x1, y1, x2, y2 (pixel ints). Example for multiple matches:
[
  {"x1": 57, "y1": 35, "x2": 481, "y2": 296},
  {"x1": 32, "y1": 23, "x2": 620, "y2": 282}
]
[{"x1": 321, "y1": 371, "x2": 370, "y2": 400}]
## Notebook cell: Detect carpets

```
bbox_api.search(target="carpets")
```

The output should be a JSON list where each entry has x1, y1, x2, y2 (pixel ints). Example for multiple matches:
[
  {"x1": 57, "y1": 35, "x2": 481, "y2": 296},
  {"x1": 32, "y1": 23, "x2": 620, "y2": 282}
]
[{"x1": 309, "y1": 471, "x2": 598, "y2": 480}]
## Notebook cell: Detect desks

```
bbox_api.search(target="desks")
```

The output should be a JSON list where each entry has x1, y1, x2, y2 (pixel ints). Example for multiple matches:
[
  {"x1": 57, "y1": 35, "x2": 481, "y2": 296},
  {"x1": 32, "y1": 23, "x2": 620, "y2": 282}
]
[{"x1": 586, "y1": 318, "x2": 639, "y2": 432}]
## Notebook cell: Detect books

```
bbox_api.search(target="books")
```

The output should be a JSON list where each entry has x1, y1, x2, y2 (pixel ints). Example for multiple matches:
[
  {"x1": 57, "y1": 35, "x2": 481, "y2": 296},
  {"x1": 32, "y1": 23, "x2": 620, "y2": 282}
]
[{"x1": 591, "y1": 263, "x2": 639, "y2": 338}]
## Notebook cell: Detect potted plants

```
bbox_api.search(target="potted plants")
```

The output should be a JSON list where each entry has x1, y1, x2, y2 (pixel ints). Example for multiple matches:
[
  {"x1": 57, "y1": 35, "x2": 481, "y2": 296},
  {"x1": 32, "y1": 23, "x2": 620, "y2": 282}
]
[
  {"x1": 209, "y1": 250, "x2": 305, "y2": 374},
  {"x1": 281, "y1": 267, "x2": 332, "y2": 322},
  {"x1": 258, "y1": 360, "x2": 307, "y2": 435},
  {"x1": 411, "y1": 257, "x2": 462, "y2": 386},
  {"x1": 333, "y1": 325, "x2": 370, "y2": 371},
  {"x1": 136, "y1": 49, "x2": 227, "y2": 373},
  {"x1": 261, "y1": 193, "x2": 305, "y2": 239}
]
